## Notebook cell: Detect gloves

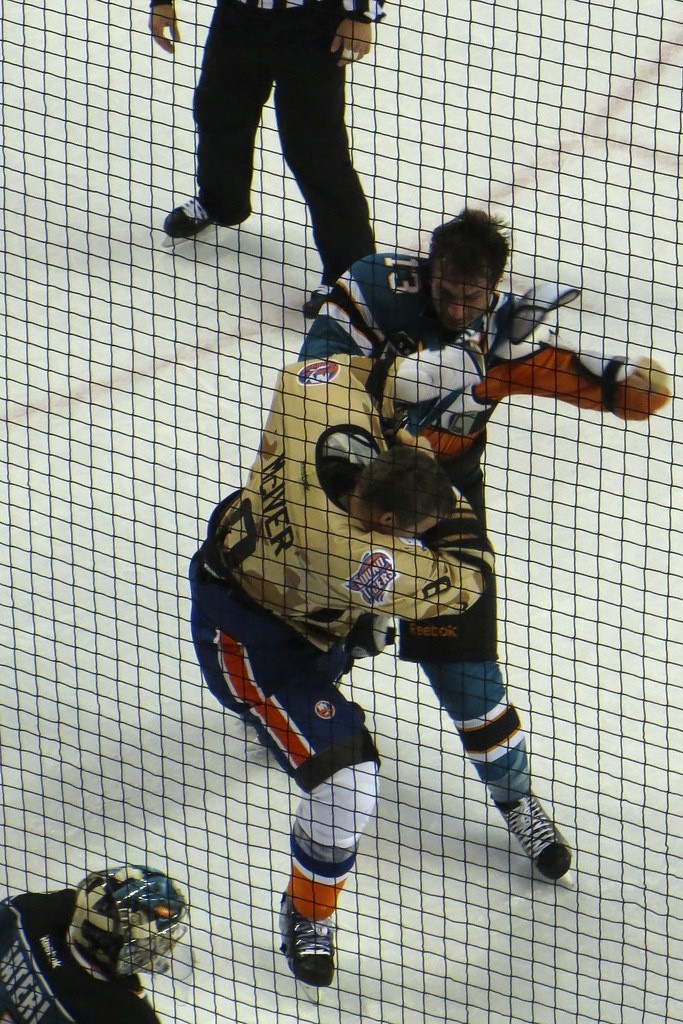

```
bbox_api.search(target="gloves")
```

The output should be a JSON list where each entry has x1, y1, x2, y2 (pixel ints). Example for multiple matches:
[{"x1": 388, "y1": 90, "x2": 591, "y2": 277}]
[{"x1": 395, "y1": 347, "x2": 486, "y2": 404}]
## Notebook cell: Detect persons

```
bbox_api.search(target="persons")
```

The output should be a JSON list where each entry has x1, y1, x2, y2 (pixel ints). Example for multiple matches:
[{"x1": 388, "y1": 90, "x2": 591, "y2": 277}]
[
  {"x1": 149, "y1": 0, "x2": 385, "y2": 316},
  {"x1": 189, "y1": 333, "x2": 495, "y2": 989},
  {"x1": 1, "y1": 865, "x2": 192, "y2": 1024},
  {"x1": 299, "y1": 211, "x2": 669, "y2": 881}
]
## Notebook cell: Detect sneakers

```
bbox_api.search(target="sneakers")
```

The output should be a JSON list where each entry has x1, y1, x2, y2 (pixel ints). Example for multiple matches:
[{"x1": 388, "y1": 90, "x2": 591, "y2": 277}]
[
  {"x1": 279, "y1": 889, "x2": 335, "y2": 988},
  {"x1": 302, "y1": 284, "x2": 333, "y2": 320},
  {"x1": 494, "y1": 790, "x2": 574, "y2": 886},
  {"x1": 162, "y1": 196, "x2": 215, "y2": 248}
]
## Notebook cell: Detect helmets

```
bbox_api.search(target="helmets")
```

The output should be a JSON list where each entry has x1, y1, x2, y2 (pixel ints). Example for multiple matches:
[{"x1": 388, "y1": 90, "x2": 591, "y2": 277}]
[{"x1": 66, "y1": 864, "x2": 187, "y2": 988}]
